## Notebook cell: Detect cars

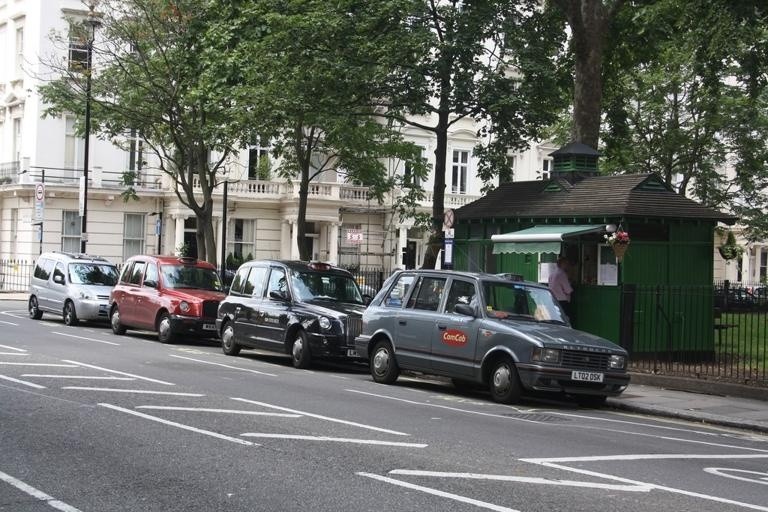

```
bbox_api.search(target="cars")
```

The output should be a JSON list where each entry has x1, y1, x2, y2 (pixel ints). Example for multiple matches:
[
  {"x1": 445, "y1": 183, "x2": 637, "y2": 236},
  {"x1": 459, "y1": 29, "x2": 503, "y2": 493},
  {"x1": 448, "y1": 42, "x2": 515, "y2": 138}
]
[
  {"x1": 216, "y1": 258, "x2": 370, "y2": 369},
  {"x1": 107, "y1": 253, "x2": 228, "y2": 345},
  {"x1": 27, "y1": 251, "x2": 121, "y2": 329},
  {"x1": 715, "y1": 286, "x2": 767, "y2": 308},
  {"x1": 352, "y1": 266, "x2": 630, "y2": 410}
]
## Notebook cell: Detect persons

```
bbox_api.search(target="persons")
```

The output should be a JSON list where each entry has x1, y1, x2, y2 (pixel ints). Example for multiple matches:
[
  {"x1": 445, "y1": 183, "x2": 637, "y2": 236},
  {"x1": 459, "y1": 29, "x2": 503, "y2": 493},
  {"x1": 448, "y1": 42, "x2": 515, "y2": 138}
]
[{"x1": 548, "y1": 258, "x2": 574, "y2": 321}]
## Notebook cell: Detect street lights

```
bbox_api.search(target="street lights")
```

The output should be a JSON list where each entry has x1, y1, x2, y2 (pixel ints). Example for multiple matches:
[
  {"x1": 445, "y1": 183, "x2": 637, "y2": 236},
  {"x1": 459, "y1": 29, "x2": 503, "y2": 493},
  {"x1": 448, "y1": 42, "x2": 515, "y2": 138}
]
[{"x1": 79, "y1": 1, "x2": 104, "y2": 257}]
